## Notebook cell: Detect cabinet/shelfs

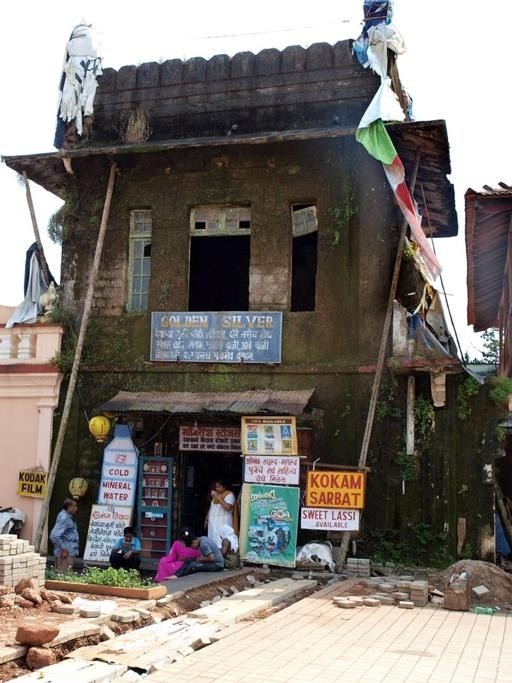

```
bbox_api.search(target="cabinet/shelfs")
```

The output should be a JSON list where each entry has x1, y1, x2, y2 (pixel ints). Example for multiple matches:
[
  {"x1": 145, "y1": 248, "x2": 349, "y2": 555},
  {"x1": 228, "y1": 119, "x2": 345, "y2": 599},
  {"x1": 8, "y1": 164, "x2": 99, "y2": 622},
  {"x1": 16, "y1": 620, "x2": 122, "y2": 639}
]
[{"x1": 135, "y1": 455, "x2": 185, "y2": 560}]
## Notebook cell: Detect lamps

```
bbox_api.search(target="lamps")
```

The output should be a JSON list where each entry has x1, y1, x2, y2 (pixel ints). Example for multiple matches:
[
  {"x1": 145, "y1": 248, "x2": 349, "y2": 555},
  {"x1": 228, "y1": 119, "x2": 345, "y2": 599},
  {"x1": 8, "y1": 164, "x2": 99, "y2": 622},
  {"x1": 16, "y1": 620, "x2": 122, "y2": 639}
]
[
  {"x1": 192, "y1": 418, "x2": 199, "y2": 431},
  {"x1": 68, "y1": 473, "x2": 90, "y2": 502},
  {"x1": 88, "y1": 410, "x2": 111, "y2": 444}
]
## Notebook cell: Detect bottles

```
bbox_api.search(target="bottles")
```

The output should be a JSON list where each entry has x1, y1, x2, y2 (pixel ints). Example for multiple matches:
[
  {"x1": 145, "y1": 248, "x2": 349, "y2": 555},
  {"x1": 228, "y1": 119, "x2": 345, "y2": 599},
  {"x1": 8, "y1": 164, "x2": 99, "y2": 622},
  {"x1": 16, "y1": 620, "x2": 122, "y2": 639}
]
[
  {"x1": 474, "y1": 606, "x2": 493, "y2": 614},
  {"x1": 454, "y1": 572, "x2": 467, "y2": 583},
  {"x1": 99, "y1": 424, "x2": 141, "y2": 506}
]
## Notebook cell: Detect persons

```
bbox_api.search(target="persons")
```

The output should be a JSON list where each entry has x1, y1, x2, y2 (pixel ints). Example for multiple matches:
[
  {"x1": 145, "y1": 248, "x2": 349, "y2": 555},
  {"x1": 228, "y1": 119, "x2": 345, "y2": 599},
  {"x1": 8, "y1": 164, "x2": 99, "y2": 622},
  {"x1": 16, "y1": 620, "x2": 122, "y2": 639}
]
[
  {"x1": 49, "y1": 498, "x2": 80, "y2": 570},
  {"x1": 153, "y1": 528, "x2": 201, "y2": 583},
  {"x1": 164, "y1": 533, "x2": 226, "y2": 578},
  {"x1": 204, "y1": 479, "x2": 239, "y2": 555},
  {"x1": 109, "y1": 527, "x2": 142, "y2": 571}
]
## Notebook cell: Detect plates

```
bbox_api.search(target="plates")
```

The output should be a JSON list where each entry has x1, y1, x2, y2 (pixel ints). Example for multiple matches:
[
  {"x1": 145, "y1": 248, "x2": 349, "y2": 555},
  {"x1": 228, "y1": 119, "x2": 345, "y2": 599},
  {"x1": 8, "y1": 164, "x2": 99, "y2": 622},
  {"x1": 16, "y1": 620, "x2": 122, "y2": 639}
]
[
  {"x1": 161, "y1": 465, "x2": 167, "y2": 471},
  {"x1": 144, "y1": 463, "x2": 149, "y2": 471}
]
